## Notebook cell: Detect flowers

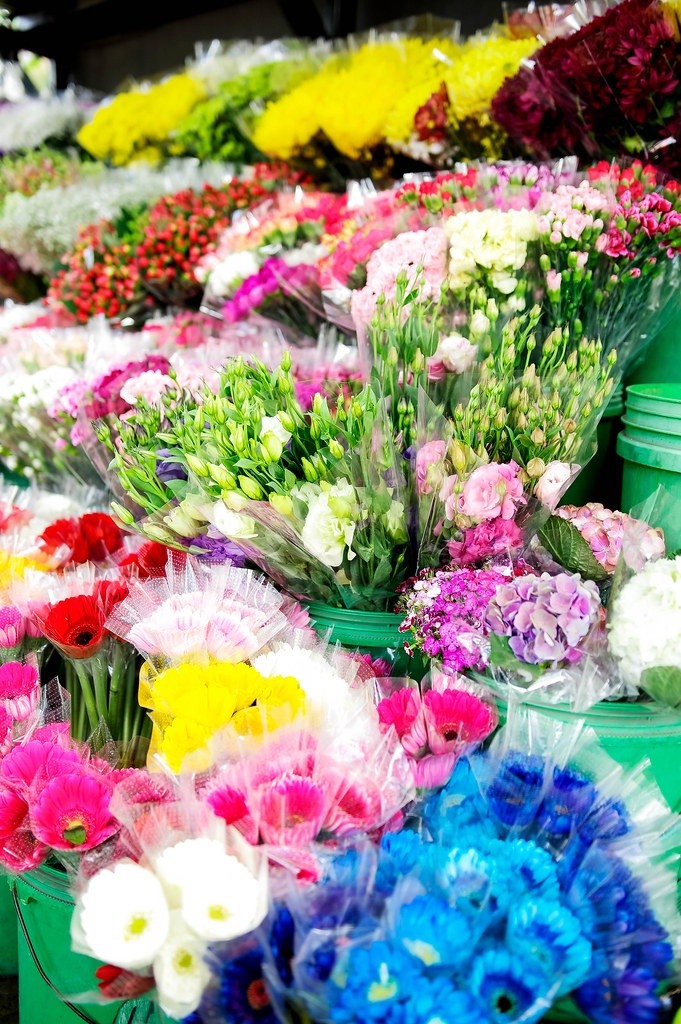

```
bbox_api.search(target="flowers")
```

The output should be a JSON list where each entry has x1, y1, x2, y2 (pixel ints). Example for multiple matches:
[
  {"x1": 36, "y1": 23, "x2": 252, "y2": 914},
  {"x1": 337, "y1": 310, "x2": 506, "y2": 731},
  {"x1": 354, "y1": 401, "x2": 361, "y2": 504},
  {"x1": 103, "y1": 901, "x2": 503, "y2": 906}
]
[{"x1": 1, "y1": 0, "x2": 681, "y2": 1024}]
